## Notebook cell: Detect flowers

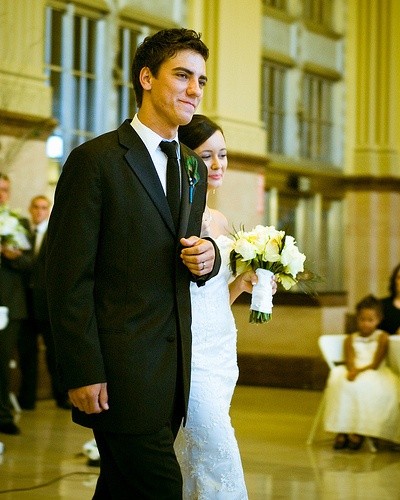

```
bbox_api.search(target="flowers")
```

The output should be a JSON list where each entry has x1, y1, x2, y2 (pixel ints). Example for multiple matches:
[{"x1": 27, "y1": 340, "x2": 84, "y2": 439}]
[
  {"x1": 225, "y1": 223, "x2": 322, "y2": 324},
  {"x1": 0, "y1": 209, "x2": 33, "y2": 252}
]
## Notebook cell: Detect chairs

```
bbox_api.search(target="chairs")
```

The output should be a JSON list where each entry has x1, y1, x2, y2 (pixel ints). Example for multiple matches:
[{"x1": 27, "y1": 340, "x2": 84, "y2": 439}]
[{"x1": 307, "y1": 334, "x2": 380, "y2": 454}]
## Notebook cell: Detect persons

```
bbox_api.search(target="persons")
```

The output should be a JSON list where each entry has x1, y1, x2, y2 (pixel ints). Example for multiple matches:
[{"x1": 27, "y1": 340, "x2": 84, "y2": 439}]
[
  {"x1": 0, "y1": 173, "x2": 73, "y2": 436},
  {"x1": 42, "y1": 28, "x2": 221, "y2": 500},
  {"x1": 323, "y1": 295, "x2": 400, "y2": 450},
  {"x1": 177, "y1": 114, "x2": 277, "y2": 500},
  {"x1": 376, "y1": 264, "x2": 400, "y2": 335}
]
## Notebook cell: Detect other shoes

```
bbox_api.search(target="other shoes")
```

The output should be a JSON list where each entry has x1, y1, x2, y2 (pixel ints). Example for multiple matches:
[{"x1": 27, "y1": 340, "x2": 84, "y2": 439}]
[
  {"x1": 349, "y1": 434, "x2": 365, "y2": 451},
  {"x1": 56, "y1": 398, "x2": 72, "y2": 410},
  {"x1": 334, "y1": 434, "x2": 348, "y2": 449},
  {"x1": 0, "y1": 420, "x2": 20, "y2": 435}
]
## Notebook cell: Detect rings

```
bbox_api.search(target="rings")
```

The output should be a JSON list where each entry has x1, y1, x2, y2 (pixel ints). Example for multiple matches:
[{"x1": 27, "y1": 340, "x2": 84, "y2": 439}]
[{"x1": 202, "y1": 262, "x2": 206, "y2": 270}]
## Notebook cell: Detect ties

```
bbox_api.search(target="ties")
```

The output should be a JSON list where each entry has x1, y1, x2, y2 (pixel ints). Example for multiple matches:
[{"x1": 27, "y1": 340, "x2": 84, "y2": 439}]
[
  {"x1": 159, "y1": 140, "x2": 180, "y2": 233},
  {"x1": 32, "y1": 229, "x2": 38, "y2": 254}
]
